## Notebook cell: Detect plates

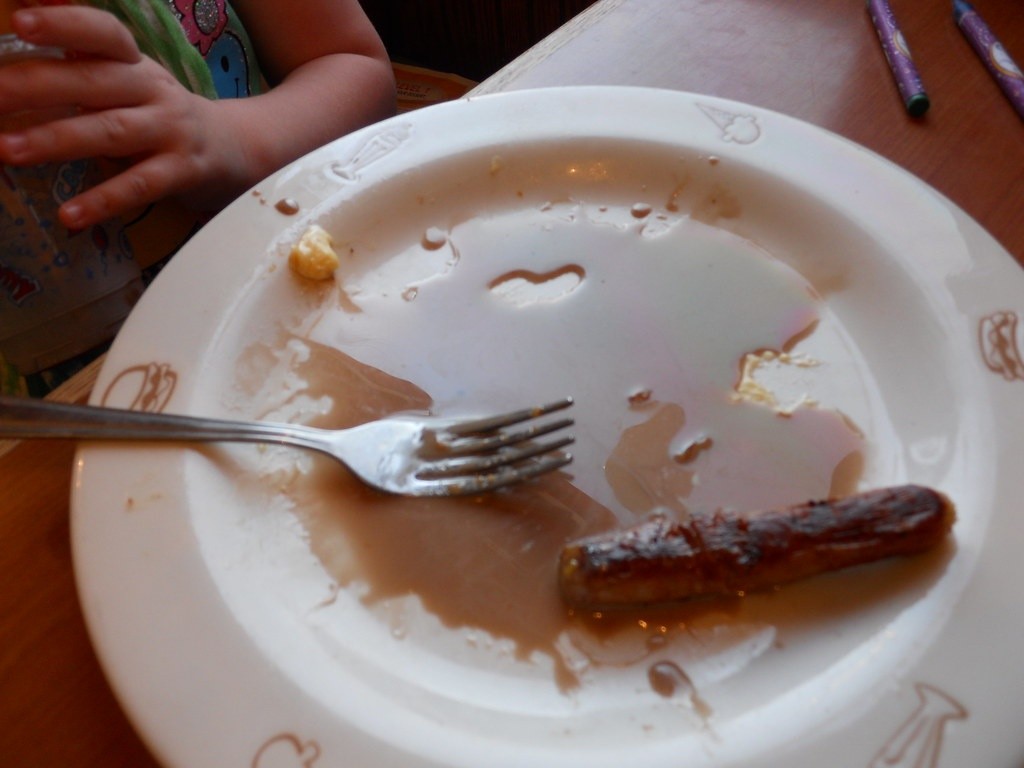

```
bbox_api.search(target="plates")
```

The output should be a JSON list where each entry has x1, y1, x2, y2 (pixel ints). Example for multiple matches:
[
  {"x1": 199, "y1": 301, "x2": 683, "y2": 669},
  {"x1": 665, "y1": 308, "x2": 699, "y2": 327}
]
[{"x1": 66, "y1": 85, "x2": 1024, "y2": 768}]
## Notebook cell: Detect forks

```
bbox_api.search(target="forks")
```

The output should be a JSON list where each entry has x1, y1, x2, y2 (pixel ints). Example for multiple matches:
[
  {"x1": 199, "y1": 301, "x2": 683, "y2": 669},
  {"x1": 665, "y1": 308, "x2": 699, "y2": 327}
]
[{"x1": 0, "y1": 392, "x2": 579, "y2": 499}]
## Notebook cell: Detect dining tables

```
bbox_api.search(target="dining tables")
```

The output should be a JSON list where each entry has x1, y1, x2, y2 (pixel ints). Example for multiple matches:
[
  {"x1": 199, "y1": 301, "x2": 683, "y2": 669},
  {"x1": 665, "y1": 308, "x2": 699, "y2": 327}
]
[{"x1": 2, "y1": 1, "x2": 1023, "y2": 766}]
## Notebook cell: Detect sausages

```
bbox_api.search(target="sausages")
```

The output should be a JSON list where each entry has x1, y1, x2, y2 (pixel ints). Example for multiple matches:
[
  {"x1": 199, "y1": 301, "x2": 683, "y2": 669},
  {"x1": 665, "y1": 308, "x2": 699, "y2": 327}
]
[{"x1": 558, "y1": 485, "x2": 955, "y2": 607}]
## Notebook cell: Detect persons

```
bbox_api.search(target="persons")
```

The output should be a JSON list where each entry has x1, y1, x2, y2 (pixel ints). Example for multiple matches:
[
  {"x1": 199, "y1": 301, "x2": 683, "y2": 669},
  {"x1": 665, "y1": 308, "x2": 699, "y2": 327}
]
[{"x1": 0, "y1": 0, "x2": 399, "y2": 398}]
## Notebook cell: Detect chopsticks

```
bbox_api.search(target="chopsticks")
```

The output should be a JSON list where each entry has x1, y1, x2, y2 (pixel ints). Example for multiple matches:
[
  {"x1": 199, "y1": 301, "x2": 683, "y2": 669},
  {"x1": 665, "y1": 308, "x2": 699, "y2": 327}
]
[{"x1": 862, "y1": 0, "x2": 1024, "y2": 116}]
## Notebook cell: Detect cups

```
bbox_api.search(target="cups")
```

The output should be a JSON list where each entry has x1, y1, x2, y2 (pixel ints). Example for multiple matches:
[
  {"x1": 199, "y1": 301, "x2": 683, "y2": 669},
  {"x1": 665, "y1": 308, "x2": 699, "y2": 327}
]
[{"x1": 0, "y1": 29, "x2": 145, "y2": 377}]
